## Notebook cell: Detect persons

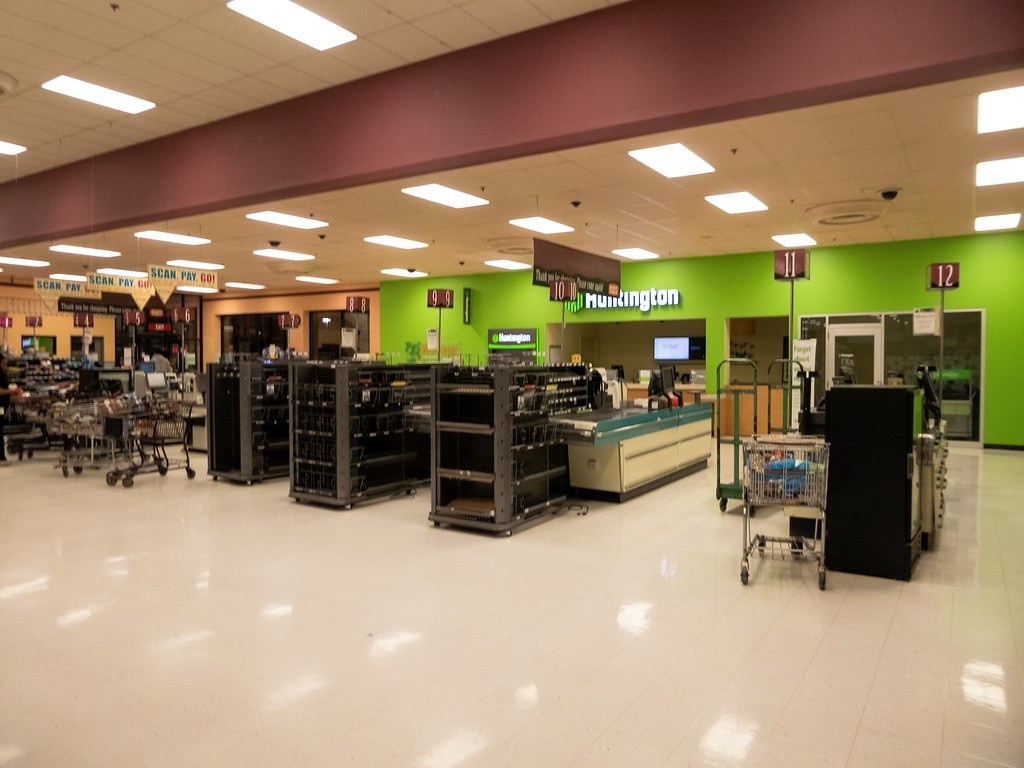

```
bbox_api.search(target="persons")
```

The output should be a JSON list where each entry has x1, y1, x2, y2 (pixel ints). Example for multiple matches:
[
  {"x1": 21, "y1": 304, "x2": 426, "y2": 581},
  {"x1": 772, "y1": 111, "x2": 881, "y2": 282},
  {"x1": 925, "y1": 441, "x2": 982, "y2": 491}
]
[
  {"x1": 150, "y1": 347, "x2": 171, "y2": 375},
  {"x1": 0, "y1": 352, "x2": 24, "y2": 466}
]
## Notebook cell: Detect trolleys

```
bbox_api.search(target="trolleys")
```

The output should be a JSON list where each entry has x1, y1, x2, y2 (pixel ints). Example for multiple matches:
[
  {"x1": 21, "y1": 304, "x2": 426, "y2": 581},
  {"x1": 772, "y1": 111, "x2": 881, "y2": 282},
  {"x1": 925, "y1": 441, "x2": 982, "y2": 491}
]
[
  {"x1": 740, "y1": 434, "x2": 830, "y2": 591},
  {"x1": 44, "y1": 394, "x2": 196, "y2": 489}
]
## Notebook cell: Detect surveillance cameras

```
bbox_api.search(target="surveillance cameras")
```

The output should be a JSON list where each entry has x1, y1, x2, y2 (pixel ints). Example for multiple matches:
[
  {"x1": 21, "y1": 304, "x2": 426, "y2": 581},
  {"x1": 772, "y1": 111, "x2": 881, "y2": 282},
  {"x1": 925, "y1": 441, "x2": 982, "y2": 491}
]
[
  {"x1": 270, "y1": 241, "x2": 280, "y2": 247},
  {"x1": 460, "y1": 262, "x2": 464, "y2": 265},
  {"x1": 220, "y1": 289, "x2": 225, "y2": 293},
  {"x1": 570, "y1": 202, "x2": 581, "y2": 207},
  {"x1": 407, "y1": 269, "x2": 415, "y2": 272},
  {"x1": 881, "y1": 191, "x2": 898, "y2": 200},
  {"x1": 319, "y1": 235, "x2": 325, "y2": 239}
]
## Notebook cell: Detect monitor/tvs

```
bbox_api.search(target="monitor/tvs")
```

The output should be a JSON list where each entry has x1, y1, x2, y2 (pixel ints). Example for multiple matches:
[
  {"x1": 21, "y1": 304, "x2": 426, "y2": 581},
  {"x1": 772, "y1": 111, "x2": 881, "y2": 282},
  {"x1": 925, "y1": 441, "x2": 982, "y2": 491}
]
[
  {"x1": 138, "y1": 362, "x2": 155, "y2": 374},
  {"x1": 652, "y1": 337, "x2": 691, "y2": 361},
  {"x1": 660, "y1": 365, "x2": 675, "y2": 393},
  {"x1": 916, "y1": 365, "x2": 940, "y2": 404}
]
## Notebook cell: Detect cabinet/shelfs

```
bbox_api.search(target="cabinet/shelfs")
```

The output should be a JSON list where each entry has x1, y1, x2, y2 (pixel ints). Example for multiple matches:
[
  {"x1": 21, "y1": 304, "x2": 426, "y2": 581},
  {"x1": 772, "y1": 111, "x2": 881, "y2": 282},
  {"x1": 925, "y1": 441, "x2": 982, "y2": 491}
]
[
  {"x1": 287, "y1": 361, "x2": 451, "y2": 508},
  {"x1": 202, "y1": 360, "x2": 308, "y2": 485},
  {"x1": 823, "y1": 383, "x2": 925, "y2": 578},
  {"x1": 426, "y1": 360, "x2": 590, "y2": 534}
]
